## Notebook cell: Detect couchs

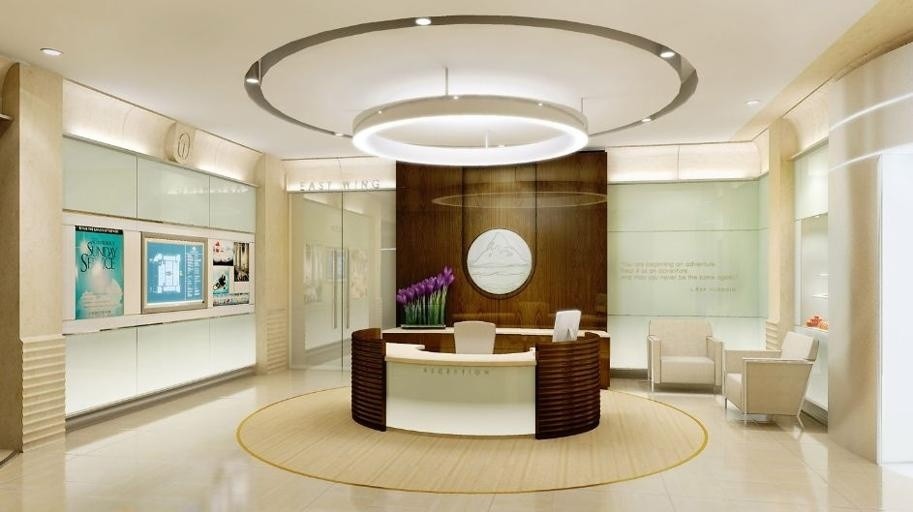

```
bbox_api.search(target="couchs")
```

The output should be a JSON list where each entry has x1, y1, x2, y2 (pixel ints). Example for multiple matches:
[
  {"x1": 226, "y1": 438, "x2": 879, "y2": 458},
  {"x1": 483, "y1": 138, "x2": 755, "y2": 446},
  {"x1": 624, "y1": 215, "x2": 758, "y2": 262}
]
[
  {"x1": 722, "y1": 332, "x2": 819, "y2": 425},
  {"x1": 647, "y1": 320, "x2": 725, "y2": 393}
]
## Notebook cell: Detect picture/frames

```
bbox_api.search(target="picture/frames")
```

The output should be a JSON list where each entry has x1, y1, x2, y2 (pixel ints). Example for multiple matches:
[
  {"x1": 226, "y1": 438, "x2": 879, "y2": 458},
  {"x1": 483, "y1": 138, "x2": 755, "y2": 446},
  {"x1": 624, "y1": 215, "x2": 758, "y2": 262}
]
[
  {"x1": 140, "y1": 231, "x2": 208, "y2": 315},
  {"x1": 207, "y1": 239, "x2": 254, "y2": 310},
  {"x1": 74, "y1": 225, "x2": 123, "y2": 320}
]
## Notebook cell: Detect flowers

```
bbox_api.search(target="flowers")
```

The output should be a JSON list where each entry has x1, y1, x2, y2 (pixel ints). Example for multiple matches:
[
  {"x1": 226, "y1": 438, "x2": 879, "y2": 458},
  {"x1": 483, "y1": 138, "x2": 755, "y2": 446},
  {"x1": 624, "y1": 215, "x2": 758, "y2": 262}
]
[{"x1": 395, "y1": 265, "x2": 454, "y2": 324}]
